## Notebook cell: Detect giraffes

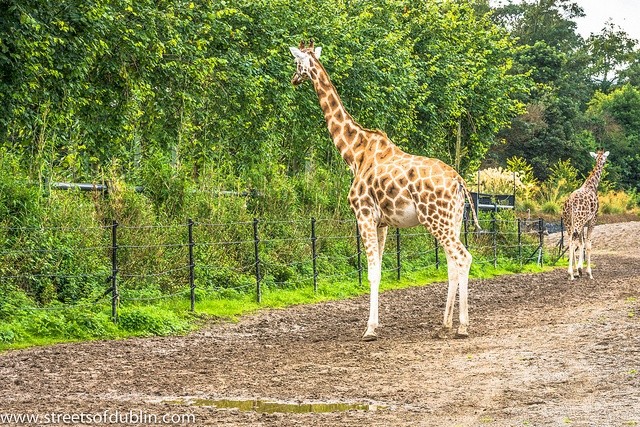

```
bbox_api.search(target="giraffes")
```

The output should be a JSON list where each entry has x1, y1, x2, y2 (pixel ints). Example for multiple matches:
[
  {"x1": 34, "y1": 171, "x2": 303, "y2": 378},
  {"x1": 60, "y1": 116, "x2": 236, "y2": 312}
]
[
  {"x1": 289, "y1": 38, "x2": 482, "y2": 342},
  {"x1": 562, "y1": 146, "x2": 610, "y2": 282}
]
